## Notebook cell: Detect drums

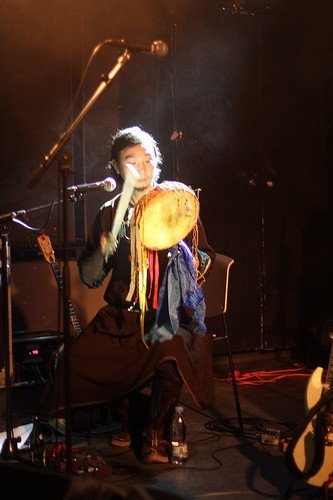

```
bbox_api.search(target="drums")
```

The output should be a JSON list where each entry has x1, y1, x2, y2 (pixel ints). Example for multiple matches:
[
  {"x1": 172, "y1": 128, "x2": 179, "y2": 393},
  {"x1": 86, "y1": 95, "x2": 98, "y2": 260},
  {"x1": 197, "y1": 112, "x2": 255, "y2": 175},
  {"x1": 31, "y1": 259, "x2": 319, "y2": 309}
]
[{"x1": 132, "y1": 181, "x2": 200, "y2": 252}]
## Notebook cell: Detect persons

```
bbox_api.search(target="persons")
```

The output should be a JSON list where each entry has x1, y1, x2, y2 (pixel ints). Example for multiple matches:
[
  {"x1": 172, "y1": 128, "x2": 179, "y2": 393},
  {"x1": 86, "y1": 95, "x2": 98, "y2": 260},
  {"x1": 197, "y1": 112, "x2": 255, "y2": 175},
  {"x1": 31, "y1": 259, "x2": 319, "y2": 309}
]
[{"x1": 53, "y1": 125, "x2": 218, "y2": 462}]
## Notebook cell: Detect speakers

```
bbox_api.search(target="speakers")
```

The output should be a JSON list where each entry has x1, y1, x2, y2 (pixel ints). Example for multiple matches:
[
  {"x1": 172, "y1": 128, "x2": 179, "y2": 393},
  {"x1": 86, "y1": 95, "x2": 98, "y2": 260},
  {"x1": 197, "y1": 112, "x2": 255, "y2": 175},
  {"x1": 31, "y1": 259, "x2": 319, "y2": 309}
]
[{"x1": 0, "y1": 462, "x2": 127, "y2": 500}]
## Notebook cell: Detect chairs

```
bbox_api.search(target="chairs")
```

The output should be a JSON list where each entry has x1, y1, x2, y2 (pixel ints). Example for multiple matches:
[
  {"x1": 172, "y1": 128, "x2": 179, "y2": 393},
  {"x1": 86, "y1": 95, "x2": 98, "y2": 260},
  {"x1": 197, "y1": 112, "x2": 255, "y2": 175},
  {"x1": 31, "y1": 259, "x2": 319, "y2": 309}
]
[{"x1": 176, "y1": 252, "x2": 244, "y2": 431}]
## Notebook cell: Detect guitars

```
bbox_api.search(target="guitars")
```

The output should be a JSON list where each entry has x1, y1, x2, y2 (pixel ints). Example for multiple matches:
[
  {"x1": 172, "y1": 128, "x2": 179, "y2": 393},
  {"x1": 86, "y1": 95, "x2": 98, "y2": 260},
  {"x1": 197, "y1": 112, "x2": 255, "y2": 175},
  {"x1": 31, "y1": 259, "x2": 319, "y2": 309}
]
[
  {"x1": 36, "y1": 229, "x2": 83, "y2": 337},
  {"x1": 291, "y1": 339, "x2": 333, "y2": 488}
]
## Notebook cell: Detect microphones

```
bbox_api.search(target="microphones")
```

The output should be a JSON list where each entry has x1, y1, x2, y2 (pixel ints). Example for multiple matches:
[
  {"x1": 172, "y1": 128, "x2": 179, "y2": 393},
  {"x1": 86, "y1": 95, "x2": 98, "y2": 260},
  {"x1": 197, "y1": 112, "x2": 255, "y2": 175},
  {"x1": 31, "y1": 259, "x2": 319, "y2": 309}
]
[
  {"x1": 105, "y1": 40, "x2": 169, "y2": 58},
  {"x1": 68, "y1": 177, "x2": 117, "y2": 192}
]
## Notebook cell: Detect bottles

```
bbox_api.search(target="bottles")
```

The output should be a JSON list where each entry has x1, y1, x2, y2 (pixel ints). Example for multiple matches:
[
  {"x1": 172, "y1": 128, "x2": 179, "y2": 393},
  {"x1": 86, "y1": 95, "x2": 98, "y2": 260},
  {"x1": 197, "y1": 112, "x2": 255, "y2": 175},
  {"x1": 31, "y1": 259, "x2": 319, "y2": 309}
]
[{"x1": 169, "y1": 405, "x2": 190, "y2": 466}]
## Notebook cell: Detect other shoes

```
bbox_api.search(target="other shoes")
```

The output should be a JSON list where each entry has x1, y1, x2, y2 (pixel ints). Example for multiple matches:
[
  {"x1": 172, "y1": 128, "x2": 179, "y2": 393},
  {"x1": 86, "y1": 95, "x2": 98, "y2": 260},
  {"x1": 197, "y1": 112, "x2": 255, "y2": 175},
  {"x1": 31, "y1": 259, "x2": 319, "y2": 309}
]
[
  {"x1": 141, "y1": 436, "x2": 170, "y2": 465},
  {"x1": 111, "y1": 417, "x2": 132, "y2": 448}
]
